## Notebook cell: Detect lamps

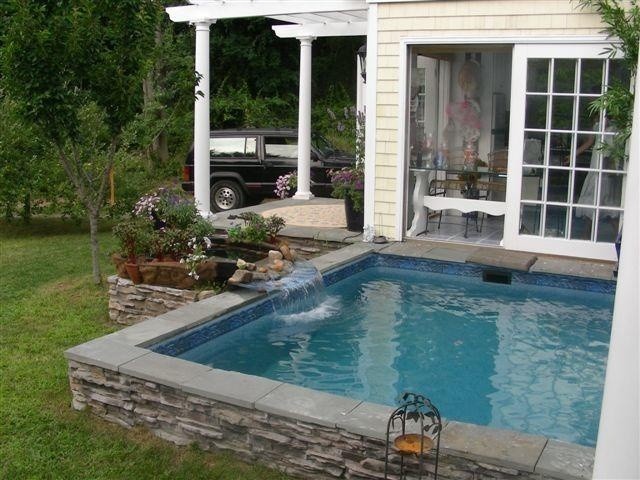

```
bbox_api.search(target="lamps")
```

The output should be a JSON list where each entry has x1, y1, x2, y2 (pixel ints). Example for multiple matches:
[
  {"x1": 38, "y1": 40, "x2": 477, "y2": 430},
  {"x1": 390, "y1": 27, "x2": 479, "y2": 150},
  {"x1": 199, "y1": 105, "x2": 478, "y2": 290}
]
[{"x1": 357, "y1": 45, "x2": 366, "y2": 85}]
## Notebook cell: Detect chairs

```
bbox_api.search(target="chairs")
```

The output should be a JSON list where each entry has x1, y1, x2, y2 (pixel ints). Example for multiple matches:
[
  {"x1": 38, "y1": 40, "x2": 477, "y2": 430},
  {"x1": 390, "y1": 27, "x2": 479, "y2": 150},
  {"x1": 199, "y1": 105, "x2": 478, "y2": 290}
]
[
  {"x1": 429, "y1": 147, "x2": 480, "y2": 236},
  {"x1": 463, "y1": 149, "x2": 509, "y2": 240}
]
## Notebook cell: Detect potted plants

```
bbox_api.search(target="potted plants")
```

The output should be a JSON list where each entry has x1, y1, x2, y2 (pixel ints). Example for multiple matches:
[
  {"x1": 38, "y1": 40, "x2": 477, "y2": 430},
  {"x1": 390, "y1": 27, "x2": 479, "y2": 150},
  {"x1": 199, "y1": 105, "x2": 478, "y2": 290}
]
[{"x1": 111, "y1": 185, "x2": 218, "y2": 289}]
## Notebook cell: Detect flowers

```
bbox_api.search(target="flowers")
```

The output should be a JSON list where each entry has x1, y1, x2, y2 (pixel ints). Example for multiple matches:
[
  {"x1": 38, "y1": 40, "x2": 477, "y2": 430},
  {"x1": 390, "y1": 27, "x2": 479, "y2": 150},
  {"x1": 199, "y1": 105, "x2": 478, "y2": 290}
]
[
  {"x1": 272, "y1": 170, "x2": 315, "y2": 200},
  {"x1": 324, "y1": 105, "x2": 366, "y2": 213}
]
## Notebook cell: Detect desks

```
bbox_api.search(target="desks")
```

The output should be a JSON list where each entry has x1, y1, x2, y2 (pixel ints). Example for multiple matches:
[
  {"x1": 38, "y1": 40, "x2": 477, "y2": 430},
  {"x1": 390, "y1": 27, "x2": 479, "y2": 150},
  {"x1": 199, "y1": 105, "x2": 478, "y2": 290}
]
[{"x1": 407, "y1": 164, "x2": 544, "y2": 240}]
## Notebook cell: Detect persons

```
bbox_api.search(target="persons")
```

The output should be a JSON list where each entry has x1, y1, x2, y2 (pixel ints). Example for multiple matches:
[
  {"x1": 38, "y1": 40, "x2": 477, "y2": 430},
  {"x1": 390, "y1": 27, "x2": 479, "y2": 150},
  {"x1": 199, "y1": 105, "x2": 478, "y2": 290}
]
[{"x1": 563, "y1": 110, "x2": 624, "y2": 239}]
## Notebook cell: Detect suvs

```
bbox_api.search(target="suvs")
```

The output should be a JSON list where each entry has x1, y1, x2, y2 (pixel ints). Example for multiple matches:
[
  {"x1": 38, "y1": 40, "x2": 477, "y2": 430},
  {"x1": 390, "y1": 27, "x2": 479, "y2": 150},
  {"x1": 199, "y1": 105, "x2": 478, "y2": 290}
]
[{"x1": 181, "y1": 129, "x2": 357, "y2": 212}]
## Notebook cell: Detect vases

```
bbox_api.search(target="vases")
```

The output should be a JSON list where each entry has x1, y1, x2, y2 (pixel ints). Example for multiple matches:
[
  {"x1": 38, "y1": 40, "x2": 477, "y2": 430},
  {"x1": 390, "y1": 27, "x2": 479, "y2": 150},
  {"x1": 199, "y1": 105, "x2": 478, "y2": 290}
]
[{"x1": 343, "y1": 189, "x2": 365, "y2": 234}]
